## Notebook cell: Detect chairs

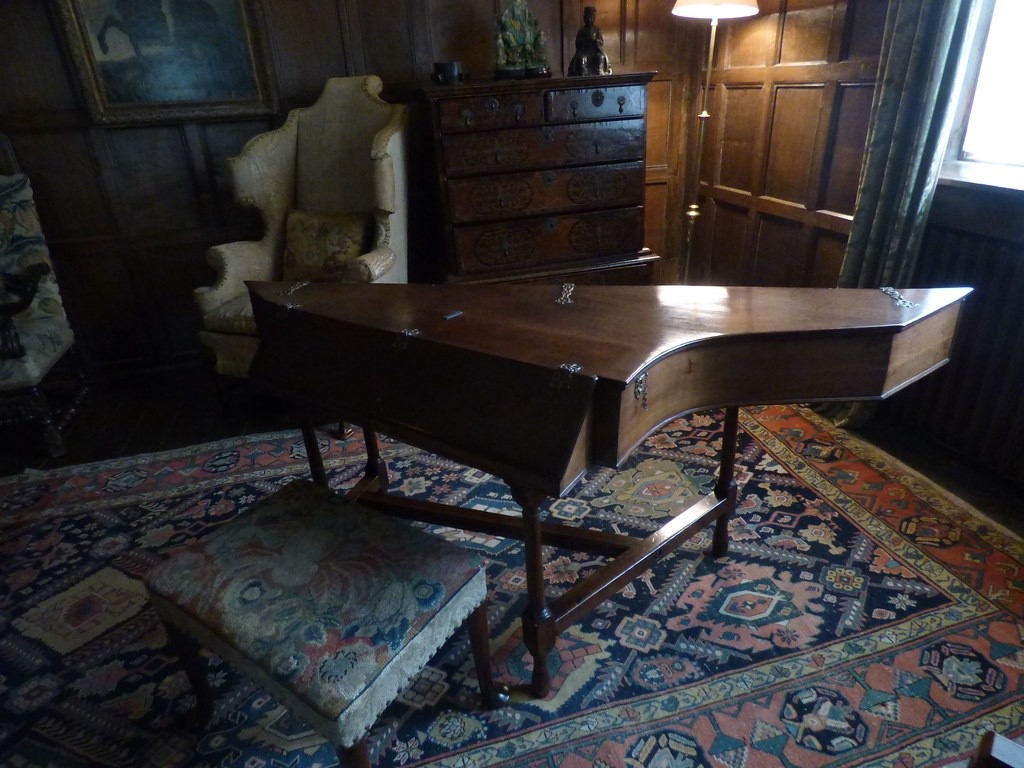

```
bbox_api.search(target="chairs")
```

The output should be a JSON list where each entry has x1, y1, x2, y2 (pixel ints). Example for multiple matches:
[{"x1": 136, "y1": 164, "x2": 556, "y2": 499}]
[
  {"x1": 0, "y1": 172, "x2": 94, "y2": 457},
  {"x1": 193, "y1": 75, "x2": 411, "y2": 443}
]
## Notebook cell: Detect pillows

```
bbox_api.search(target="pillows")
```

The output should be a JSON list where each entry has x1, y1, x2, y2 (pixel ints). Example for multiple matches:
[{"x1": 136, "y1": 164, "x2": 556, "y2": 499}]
[{"x1": 283, "y1": 209, "x2": 366, "y2": 282}]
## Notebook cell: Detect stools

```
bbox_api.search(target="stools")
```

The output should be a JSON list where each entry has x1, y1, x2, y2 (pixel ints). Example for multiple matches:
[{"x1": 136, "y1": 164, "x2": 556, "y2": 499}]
[{"x1": 143, "y1": 478, "x2": 502, "y2": 768}]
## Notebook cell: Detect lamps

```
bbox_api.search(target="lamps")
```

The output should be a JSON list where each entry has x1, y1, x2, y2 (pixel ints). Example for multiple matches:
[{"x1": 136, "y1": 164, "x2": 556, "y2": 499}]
[{"x1": 670, "y1": 0, "x2": 760, "y2": 284}]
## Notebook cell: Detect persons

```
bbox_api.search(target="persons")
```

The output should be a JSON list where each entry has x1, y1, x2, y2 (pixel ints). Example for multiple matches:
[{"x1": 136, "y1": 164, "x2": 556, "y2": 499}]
[{"x1": 572, "y1": 7, "x2": 606, "y2": 75}]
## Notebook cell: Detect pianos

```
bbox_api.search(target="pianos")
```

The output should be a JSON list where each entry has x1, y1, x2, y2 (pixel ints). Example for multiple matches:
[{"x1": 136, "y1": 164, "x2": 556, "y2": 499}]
[{"x1": 239, "y1": 263, "x2": 984, "y2": 701}]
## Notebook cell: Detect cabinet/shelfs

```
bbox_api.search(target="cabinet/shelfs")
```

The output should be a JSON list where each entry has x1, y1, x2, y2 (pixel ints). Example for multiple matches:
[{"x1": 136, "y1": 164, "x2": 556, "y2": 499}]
[{"x1": 424, "y1": 69, "x2": 659, "y2": 286}]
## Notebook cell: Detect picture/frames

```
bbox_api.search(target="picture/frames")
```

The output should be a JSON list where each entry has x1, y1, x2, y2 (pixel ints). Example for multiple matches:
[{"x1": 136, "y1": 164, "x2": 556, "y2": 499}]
[{"x1": 59, "y1": 0, "x2": 283, "y2": 128}]
